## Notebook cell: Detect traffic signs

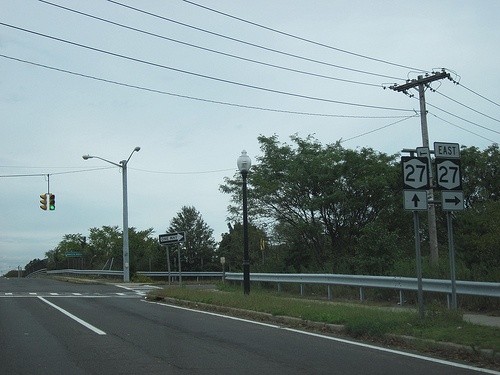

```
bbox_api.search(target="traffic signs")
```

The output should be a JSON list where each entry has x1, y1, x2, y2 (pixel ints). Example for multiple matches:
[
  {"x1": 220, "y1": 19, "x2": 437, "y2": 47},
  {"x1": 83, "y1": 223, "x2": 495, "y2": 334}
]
[{"x1": 159, "y1": 232, "x2": 186, "y2": 244}]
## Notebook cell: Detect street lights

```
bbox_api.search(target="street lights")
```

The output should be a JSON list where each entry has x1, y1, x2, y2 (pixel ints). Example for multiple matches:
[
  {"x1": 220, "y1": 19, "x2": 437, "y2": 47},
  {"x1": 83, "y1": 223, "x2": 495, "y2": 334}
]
[
  {"x1": 237, "y1": 150, "x2": 250, "y2": 296},
  {"x1": 81, "y1": 146, "x2": 141, "y2": 282}
]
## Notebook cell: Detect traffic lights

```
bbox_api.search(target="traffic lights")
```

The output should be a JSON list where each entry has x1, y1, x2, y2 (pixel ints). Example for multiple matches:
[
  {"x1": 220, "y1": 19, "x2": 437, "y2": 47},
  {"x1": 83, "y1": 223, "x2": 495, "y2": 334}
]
[
  {"x1": 39, "y1": 194, "x2": 47, "y2": 210},
  {"x1": 49, "y1": 194, "x2": 55, "y2": 210}
]
